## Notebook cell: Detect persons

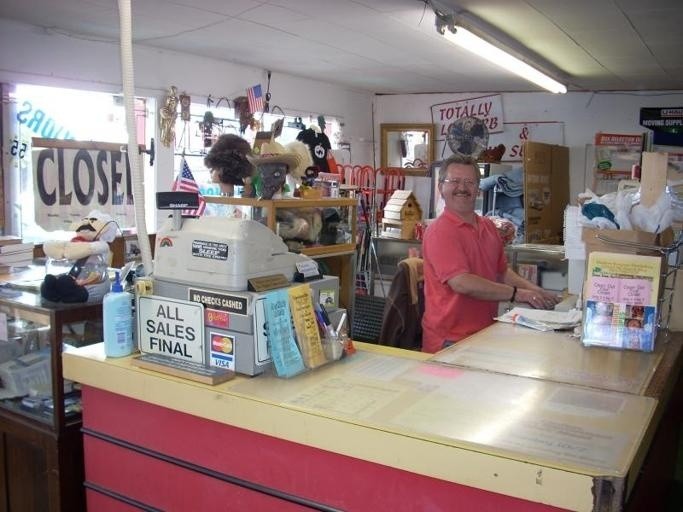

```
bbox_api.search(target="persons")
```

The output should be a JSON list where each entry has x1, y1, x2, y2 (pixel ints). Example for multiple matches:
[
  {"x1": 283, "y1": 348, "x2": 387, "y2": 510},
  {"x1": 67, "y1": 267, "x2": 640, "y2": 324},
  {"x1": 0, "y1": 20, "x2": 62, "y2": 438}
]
[
  {"x1": 40, "y1": 209, "x2": 118, "y2": 305},
  {"x1": 204, "y1": 147, "x2": 253, "y2": 195},
  {"x1": 420, "y1": 155, "x2": 560, "y2": 354}
]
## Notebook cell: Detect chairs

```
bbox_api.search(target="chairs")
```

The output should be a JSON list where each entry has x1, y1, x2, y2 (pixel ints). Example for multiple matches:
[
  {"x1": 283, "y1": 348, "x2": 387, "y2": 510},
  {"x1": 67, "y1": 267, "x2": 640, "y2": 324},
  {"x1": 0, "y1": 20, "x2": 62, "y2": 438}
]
[{"x1": 380, "y1": 257, "x2": 423, "y2": 350}]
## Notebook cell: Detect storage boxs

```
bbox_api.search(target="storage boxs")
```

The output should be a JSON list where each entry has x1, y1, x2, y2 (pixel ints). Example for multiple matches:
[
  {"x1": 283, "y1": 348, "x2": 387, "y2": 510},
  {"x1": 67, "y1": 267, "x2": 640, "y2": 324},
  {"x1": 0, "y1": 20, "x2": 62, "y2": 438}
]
[{"x1": 582, "y1": 227, "x2": 674, "y2": 323}]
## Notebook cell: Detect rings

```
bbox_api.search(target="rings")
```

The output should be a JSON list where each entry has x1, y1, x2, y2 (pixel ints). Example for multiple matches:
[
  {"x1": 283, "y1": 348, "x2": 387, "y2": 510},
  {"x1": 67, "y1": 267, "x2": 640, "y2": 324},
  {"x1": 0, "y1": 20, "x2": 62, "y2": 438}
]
[{"x1": 531, "y1": 295, "x2": 536, "y2": 300}]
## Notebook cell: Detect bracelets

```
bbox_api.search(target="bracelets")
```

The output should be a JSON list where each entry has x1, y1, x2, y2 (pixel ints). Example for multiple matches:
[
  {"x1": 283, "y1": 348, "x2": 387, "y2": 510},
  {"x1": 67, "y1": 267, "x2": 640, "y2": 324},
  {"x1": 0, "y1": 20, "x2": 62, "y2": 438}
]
[{"x1": 510, "y1": 286, "x2": 517, "y2": 303}]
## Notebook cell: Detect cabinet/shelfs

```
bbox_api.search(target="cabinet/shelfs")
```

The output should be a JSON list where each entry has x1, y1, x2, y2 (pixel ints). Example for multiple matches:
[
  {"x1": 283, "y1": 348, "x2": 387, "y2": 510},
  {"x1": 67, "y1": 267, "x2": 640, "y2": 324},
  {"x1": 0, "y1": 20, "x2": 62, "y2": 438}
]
[{"x1": 198, "y1": 197, "x2": 358, "y2": 342}]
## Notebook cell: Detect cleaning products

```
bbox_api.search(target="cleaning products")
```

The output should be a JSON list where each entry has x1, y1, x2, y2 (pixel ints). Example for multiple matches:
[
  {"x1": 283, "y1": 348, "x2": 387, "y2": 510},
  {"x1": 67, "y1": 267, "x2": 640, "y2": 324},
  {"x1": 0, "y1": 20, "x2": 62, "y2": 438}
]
[{"x1": 102, "y1": 272, "x2": 133, "y2": 357}]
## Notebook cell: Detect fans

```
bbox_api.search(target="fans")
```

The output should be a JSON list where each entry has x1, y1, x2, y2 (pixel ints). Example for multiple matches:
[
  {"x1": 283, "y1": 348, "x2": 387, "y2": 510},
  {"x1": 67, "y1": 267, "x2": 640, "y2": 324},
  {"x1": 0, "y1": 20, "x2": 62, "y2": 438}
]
[{"x1": 441, "y1": 116, "x2": 489, "y2": 160}]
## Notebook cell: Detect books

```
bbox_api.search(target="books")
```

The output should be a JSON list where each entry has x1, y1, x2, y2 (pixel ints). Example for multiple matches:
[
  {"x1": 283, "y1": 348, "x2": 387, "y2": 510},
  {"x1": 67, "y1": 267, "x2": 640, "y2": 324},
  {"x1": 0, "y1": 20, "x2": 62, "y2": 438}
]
[
  {"x1": 0, "y1": 233, "x2": 35, "y2": 270},
  {"x1": 582, "y1": 251, "x2": 661, "y2": 352}
]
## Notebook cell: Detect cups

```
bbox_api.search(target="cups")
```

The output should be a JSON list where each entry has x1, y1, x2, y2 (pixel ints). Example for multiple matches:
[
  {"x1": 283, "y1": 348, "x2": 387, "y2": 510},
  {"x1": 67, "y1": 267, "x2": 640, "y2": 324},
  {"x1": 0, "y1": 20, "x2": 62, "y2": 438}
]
[{"x1": 320, "y1": 332, "x2": 345, "y2": 360}]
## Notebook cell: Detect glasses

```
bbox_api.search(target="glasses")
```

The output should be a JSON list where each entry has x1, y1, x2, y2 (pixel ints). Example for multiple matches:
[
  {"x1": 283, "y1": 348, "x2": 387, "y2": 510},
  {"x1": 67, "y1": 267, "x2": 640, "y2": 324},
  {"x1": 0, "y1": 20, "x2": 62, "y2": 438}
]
[{"x1": 443, "y1": 178, "x2": 475, "y2": 185}]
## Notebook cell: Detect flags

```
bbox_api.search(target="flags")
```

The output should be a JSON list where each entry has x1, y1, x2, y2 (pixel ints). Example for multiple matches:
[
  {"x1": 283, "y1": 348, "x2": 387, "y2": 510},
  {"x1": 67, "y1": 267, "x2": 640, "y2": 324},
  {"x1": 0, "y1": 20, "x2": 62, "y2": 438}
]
[
  {"x1": 246, "y1": 84, "x2": 263, "y2": 115},
  {"x1": 170, "y1": 158, "x2": 207, "y2": 217}
]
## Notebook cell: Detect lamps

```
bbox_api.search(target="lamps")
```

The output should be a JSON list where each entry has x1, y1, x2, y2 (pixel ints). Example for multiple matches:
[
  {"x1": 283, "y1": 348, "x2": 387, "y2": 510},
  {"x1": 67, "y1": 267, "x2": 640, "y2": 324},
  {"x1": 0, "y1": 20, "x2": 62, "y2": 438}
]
[{"x1": 435, "y1": 14, "x2": 567, "y2": 96}]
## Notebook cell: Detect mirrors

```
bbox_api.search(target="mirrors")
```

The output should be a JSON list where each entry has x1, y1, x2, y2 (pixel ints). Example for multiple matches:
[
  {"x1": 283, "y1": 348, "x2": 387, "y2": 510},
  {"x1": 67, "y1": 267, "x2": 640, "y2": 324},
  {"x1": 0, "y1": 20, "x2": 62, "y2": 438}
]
[{"x1": 381, "y1": 123, "x2": 435, "y2": 177}]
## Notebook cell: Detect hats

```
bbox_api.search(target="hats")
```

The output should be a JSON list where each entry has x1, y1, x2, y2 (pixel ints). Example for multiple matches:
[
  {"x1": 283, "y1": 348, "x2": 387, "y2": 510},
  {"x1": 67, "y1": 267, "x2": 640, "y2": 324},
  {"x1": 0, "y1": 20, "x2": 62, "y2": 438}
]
[{"x1": 246, "y1": 143, "x2": 301, "y2": 174}]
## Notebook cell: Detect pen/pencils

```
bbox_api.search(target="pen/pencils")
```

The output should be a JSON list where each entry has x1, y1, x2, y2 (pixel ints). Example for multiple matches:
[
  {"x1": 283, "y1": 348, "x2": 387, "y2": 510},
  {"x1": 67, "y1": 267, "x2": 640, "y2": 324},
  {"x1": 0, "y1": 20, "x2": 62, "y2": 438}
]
[{"x1": 312, "y1": 301, "x2": 347, "y2": 361}]
